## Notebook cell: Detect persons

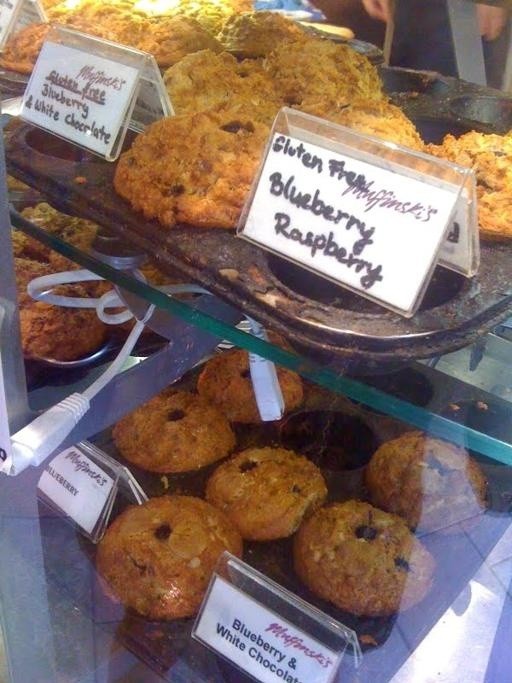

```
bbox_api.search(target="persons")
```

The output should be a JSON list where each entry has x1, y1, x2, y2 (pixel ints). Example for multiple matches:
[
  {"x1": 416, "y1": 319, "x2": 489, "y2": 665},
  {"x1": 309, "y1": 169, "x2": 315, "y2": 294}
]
[{"x1": 361, "y1": 0, "x2": 512, "y2": 96}]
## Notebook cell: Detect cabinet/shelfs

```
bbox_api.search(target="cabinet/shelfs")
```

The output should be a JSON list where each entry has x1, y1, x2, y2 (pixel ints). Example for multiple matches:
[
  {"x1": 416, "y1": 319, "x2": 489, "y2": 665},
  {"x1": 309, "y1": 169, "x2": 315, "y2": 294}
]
[{"x1": 0, "y1": 171, "x2": 512, "y2": 683}]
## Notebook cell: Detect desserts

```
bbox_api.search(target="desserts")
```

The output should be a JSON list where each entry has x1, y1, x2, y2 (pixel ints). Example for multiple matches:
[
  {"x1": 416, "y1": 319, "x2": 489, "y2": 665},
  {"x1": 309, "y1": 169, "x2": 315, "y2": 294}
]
[
  {"x1": 6, "y1": 174, "x2": 198, "y2": 360},
  {"x1": 0, "y1": 0, "x2": 512, "y2": 243}
]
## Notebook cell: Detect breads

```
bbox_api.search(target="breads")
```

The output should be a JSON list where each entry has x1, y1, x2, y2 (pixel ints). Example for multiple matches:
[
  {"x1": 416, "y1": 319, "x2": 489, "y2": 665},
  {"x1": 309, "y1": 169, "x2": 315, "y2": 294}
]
[
  {"x1": 292, "y1": 502, "x2": 434, "y2": 616},
  {"x1": 96, "y1": 496, "x2": 242, "y2": 618},
  {"x1": 207, "y1": 447, "x2": 326, "y2": 541},
  {"x1": 112, "y1": 387, "x2": 234, "y2": 473},
  {"x1": 366, "y1": 437, "x2": 488, "y2": 536},
  {"x1": 196, "y1": 349, "x2": 303, "y2": 423}
]
